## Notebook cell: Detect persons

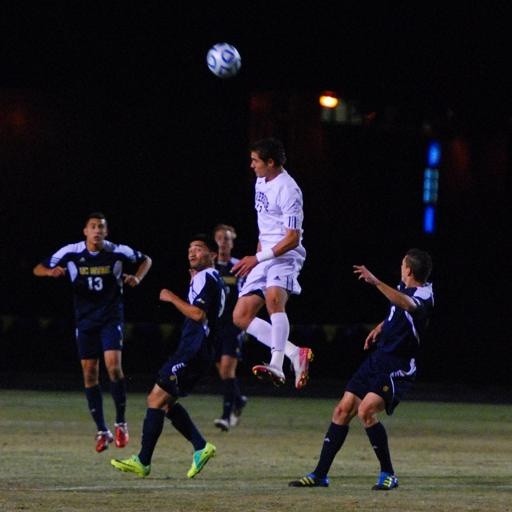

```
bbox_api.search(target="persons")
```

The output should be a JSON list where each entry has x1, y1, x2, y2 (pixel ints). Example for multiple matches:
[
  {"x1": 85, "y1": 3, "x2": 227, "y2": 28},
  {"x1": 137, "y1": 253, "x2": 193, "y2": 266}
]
[
  {"x1": 230, "y1": 137, "x2": 314, "y2": 389},
  {"x1": 289, "y1": 248, "x2": 436, "y2": 490},
  {"x1": 213, "y1": 225, "x2": 249, "y2": 432},
  {"x1": 33, "y1": 214, "x2": 152, "y2": 453},
  {"x1": 110, "y1": 234, "x2": 227, "y2": 480}
]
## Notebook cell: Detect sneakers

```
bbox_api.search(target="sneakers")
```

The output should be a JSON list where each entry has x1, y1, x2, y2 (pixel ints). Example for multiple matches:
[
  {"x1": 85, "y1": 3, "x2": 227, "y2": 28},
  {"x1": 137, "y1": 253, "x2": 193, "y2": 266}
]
[
  {"x1": 115, "y1": 421, "x2": 129, "y2": 448},
  {"x1": 230, "y1": 395, "x2": 248, "y2": 425},
  {"x1": 252, "y1": 365, "x2": 287, "y2": 388},
  {"x1": 213, "y1": 417, "x2": 230, "y2": 431},
  {"x1": 187, "y1": 442, "x2": 215, "y2": 479},
  {"x1": 96, "y1": 428, "x2": 113, "y2": 452},
  {"x1": 370, "y1": 471, "x2": 399, "y2": 490},
  {"x1": 288, "y1": 473, "x2": 328, "y2": 488},
  {"x1": 110, "y1": 455, "x2": 151, "y2": 478},
  {"x1": 292, "y1": 347, "x2": 315, "y2": 390}
]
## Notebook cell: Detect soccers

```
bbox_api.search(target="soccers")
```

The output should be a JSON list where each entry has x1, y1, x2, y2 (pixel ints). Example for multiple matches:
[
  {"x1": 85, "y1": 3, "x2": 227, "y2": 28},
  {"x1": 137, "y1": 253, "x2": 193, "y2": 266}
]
[{"x1": 207, "y1": 44, "x2": 243, "y2": 78}]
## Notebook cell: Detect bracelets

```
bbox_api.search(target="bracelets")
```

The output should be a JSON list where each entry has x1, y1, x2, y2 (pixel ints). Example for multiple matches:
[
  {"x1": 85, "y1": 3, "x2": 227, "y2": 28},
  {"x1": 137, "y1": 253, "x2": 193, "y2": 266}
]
[{"x1": 255, "y1": 247, "x2": 276, "y2": 263}]
